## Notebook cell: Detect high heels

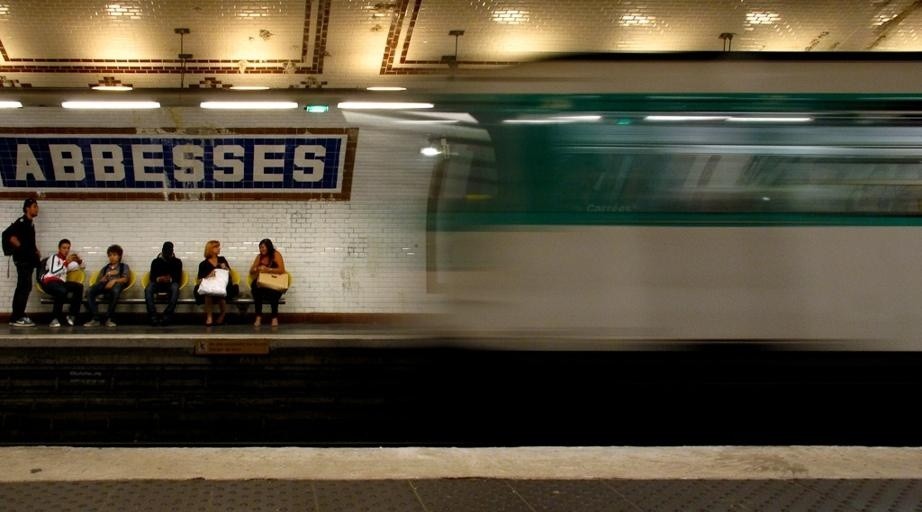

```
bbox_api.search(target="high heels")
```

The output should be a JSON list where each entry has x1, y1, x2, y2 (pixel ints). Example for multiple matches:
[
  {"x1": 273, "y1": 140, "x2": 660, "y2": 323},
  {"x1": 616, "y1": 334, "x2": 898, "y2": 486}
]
[
  {"x1": 205, "y1": 316, "x2": 225, "y2": 326},
  {"x1": 254, "y1": 317, "x2": 278, "y2": 327}
]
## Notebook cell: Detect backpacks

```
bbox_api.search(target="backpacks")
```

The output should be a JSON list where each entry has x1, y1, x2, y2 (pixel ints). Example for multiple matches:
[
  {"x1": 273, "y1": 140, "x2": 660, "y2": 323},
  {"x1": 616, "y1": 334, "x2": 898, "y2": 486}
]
[
  {"x1": 36, "y1": 257, "x2": 48, "y2": 283},
  {"x1": 2, "y1": 216, "x2": 24, "y2": 256}
]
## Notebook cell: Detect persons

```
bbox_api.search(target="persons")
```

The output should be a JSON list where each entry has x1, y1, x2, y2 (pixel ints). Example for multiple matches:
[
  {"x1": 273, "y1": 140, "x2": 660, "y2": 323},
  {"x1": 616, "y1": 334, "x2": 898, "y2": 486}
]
[
  {"x1": 7, "y1": 195, "x2": 42, "y2": 326},
  {"x1": 248, "y1": 237, "x2": 288, "y2": 328},
  {"x1": 82, "y1": 243, "x2": 131, "y2": 328},
  {"x1": 40, "y1": 236, "x2": 86, "y2": 328},
  {"x1": 144, "y1": 240, "x2": 183, "y2": 326},
  {"x1": 194, "y1": 240, "x2": 231, "y2": 327}
]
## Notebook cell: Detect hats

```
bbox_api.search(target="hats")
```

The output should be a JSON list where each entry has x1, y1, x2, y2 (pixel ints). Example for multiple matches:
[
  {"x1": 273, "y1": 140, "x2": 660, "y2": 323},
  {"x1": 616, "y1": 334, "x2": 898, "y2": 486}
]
[{"x1": 162, "y1": 242, "x2": 173, "y2": 257}]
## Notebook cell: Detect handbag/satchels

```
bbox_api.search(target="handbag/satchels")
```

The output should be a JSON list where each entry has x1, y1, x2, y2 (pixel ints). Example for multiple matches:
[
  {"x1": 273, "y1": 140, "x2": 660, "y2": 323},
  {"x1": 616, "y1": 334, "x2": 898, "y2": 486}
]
[
  {"x1": 257, "y1": 272, "x2": 289, "y2": 291},
  {"x1": 197, "y1": 268, "x2": 229, "y2": 296}
]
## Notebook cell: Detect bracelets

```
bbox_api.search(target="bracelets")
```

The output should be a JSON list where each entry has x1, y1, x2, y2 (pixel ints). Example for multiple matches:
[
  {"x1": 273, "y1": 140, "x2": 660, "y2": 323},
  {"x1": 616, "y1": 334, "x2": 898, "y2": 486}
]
[{"x1": 269, "y1": 268, "x2": 272, "y2": 272}]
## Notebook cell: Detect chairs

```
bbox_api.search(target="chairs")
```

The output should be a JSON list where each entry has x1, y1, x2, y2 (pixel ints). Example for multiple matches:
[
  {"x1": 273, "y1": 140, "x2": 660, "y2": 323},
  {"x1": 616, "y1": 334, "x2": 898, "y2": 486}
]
[{"x1": 36, "y1": 266, "x2": 292, "y2": 319}]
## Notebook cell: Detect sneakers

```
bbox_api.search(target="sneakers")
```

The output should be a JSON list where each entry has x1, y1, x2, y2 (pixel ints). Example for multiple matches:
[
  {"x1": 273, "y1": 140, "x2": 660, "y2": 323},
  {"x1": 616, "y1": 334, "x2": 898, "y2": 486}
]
[{"x1": 9, "y1": 316, "x2": 117, "y2": 328}]
